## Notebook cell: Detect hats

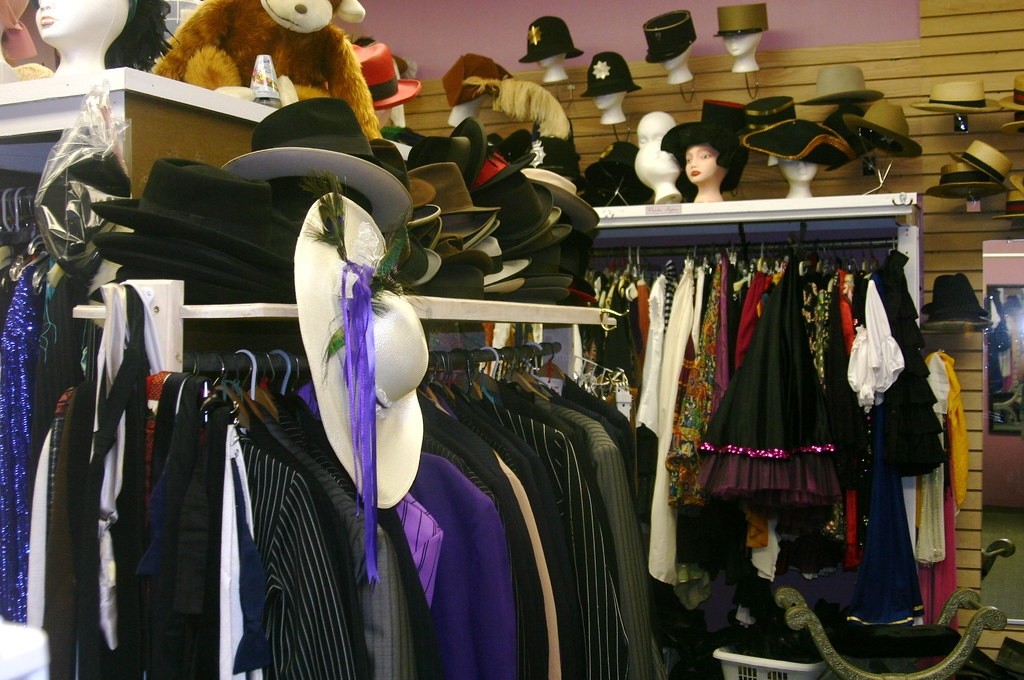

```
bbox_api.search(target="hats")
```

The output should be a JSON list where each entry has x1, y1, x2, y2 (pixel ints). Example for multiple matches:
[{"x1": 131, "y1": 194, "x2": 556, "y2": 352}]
[
  {"x1": 583, "y1": 66, "x2": 1024, "y2": 221},
  {"x1": 519, "y1": 16, "x2": 584, "y2": 63},
  {"x1": 712, "y1": 3, "x2": 768, "y2": 37},
  {"x1": 328, "y1": 43, "x2": 422, "y2": 111},
  {"x1": 643, "y1": 10, "x2": 697, "y2": 63},
  {"x1": 921, "y1": 273, "x2": 994, "y2": 331},
  {"x1": 90, "y1": 96, "x2": 600, "y2": 305},
  {"x1": 295, "y1": 170, "x2": 430, "y2": 582},
  {"x1": 580, "y1": 52, "x2": 642, "y2": 97}
]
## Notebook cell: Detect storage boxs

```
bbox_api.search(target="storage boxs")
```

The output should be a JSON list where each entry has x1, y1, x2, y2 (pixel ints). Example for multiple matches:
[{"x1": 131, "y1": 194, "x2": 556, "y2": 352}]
[{"x1": 711, "y1": 643, "x2": 828, "y2": 680}]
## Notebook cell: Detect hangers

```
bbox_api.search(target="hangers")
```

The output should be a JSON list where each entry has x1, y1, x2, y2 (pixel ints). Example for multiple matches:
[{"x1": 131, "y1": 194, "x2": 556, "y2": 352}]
[
  {"x1": 581, "y1": 236, "x2": 900, "y2": 288},
  {"x1": 0, "y1": 185, "x2": 53, "y2": 292},
  {"x1": 185, "y1": 337, "x2": 569, "y2": 438}
]
